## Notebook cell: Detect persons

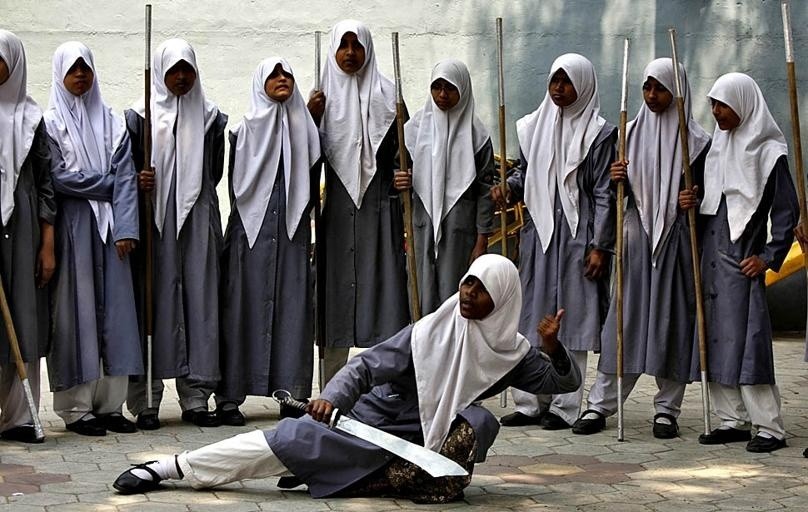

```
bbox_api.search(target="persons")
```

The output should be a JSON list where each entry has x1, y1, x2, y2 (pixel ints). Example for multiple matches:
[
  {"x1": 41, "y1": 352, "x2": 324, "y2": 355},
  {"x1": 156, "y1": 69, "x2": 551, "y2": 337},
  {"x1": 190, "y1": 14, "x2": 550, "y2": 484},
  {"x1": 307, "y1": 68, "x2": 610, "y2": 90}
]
[
  {"x1": 214, "y1": 55, "x2": 323, "y2": 427},
  {"x1": 568, "y1": 54, "x2": 712, "y2": 436},
  {"x1": 1, "y1": 27, "x2": 56, "y2": 443},
  {"x1": 111, "y1": 253, "x2": 585, "y2": 506},
  {"x1": 485, "y1": 51, "x2": 617, "y2": 431},
  {"x1": 392, "y1": 57, "x2": 496, "y2": 328},
  {"x1": 42, "y1": 39, "x2": 141, "y2": 438},
  {"x1": 307, "y1": 18, "x2": 411, "y2": 394},
  {"x1": 677, "y1": 72, "x2": 800, "y2": 452},
  {"x1": 121, "y1": 37, "x2": 230, "y2": 431}
]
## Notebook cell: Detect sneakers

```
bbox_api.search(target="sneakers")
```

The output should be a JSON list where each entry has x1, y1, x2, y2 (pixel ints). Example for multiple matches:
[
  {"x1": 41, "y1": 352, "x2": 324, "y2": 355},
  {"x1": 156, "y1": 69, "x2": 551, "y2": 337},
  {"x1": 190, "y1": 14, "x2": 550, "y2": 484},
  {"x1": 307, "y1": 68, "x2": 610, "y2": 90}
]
[{"x1": 112, "y1": 460, "x2": 164, "y2": 492}]
